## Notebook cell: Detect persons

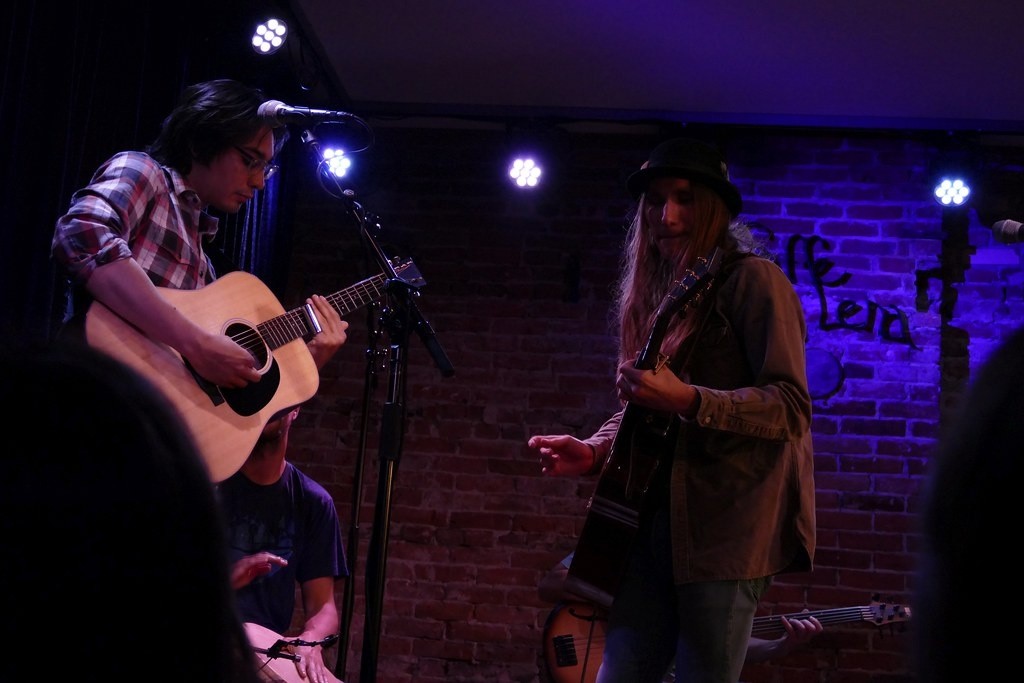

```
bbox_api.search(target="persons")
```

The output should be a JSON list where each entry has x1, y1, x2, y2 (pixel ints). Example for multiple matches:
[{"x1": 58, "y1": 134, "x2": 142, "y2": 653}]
[
  {"x1": 541, "y1": 549, "x2": 824, "y2": 683},
  {"x1": 528, "y1": 145, "x2": 816, "y2": 683},
  {"x1": 3, "y1": 74, "x2": 351, "y2": 682},
  {"x1": 208, "y1": 404, "x2": 350, "y2": 682}
]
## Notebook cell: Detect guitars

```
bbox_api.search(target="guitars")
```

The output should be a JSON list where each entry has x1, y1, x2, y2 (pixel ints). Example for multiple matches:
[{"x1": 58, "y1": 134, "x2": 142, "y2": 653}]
[
  {"x1": 83, "y1": 256, "x2": 427, "y2": 484},
  {"x1": 566, "y1": 255, "x2": 716, "y2": 610},
  {"x1": 543, "y1": 601, "x2": 911, "y2": 683}
]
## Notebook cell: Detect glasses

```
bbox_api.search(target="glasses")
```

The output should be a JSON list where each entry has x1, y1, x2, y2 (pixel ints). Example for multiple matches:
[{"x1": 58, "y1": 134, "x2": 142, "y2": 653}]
[{"x1": 232, "y1": 145, "x2": 280, "y2": 180}]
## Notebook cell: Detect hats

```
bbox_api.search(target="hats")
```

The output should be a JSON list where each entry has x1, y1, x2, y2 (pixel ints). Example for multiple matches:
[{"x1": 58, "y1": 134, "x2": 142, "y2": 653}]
[{"x1": 627, "y1": 137, "x2": 746, "y2": 222}]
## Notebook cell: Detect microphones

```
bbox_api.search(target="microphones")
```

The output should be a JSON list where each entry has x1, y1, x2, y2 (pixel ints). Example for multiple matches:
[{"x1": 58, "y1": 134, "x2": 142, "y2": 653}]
[
  {"x1": 257, "y1": 101, "x2": 354, "y2": 130},
  {"x1": 993, "y1": 219, "x2": 1023, "y2": 246}
]
